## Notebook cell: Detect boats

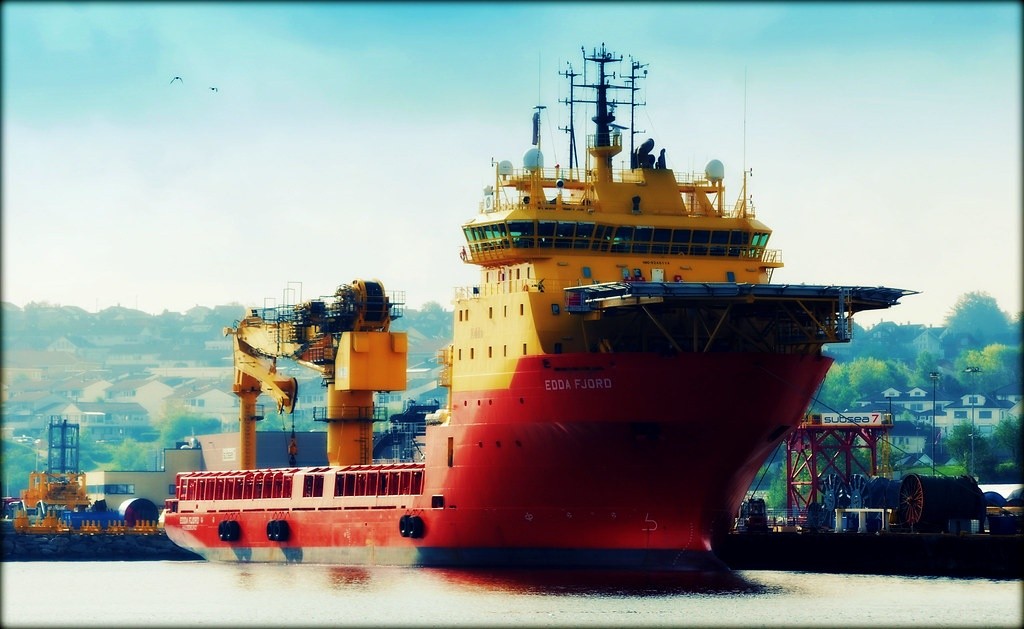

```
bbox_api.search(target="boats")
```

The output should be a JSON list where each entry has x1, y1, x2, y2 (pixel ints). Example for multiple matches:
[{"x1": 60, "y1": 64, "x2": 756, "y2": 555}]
[
  {"x1": 730, "y1": 413, "x2": 1024, "y2": 581},
  {"x1": 159, "y1": 40, "x2": 924, "y2": 567}
]
